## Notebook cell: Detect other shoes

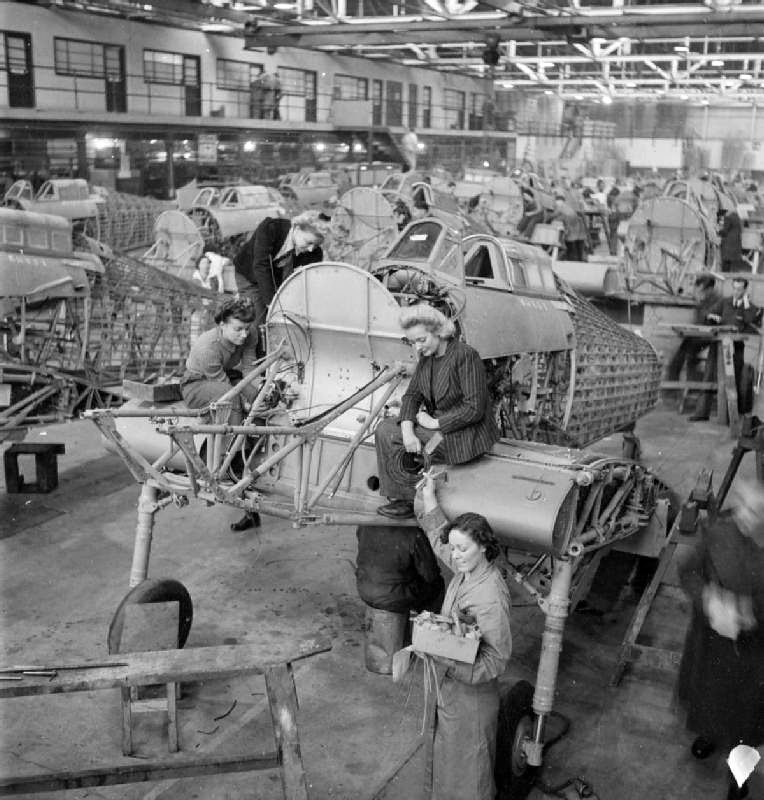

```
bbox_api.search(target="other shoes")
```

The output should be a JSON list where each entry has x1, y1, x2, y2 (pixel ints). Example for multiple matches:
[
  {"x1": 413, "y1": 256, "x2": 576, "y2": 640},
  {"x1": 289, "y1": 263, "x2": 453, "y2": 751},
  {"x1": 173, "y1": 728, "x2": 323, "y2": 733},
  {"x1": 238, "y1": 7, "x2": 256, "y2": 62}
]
[
  {"x1": 255, "y1": 344, "x2": 266, "y2": 364},
  {"x1": 230, "y1": 511, "x2": 261, "y2": 531},
  {"x1": 226, "y1": 457, "x2": 243, "y2": 484}
]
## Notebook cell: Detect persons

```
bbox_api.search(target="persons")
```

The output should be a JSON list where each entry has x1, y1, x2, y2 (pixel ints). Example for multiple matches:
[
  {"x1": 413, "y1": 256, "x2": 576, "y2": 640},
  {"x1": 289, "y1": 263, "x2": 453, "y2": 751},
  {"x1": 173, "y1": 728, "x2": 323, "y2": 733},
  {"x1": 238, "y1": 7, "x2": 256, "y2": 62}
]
[
  {"x1": 583, "y1": 178, "x2": 642, "y2": 256},
  {"x1": 716, "y1": 208, "x2": 741, "y2": 271},
  {"x1": 664, "y1": 273, "x2": 759, "y2": 422},
  {"x1": 674, "y1": 476, "x2": 764, "y2": 758},
  {"x1": 510, "y1": 189, "x2": 543, "y2": 240},
  {"x1": 181, "y1": 211, "x2": 332, "y2": 529},
  {"x1": 375, "y1": 306, "x2": 501, "y2": 519},
  {"x1": 355, "y1": 525, "x2": 446, "y2": 614},
  {"x1": 393, "y1": 202, "x2": 433, "y2": 232},
  {"x1": 545, "y1": 196, "x2": 586, "y2": 261},
  {"x1": 408, "y1": 473, "x2": 511, "y2": 800}
]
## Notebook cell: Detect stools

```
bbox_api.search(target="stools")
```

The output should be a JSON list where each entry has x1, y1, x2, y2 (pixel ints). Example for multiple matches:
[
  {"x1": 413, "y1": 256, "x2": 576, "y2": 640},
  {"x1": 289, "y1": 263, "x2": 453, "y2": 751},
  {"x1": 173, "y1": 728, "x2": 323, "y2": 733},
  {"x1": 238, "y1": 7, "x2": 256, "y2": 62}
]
[{"x1": 119, "y1": 601, "x2": 181, "y2": 756}]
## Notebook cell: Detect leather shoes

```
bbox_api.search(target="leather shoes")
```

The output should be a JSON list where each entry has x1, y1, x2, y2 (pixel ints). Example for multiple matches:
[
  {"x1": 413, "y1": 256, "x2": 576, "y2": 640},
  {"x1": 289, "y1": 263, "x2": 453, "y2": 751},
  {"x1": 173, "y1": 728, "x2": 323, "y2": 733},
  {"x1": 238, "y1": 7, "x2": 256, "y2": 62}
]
[
  {"x1": 691, "y1": 734, "x2": 716, "y2": 759},
  {"x1": 726, "y1": 776, "x2": 750, "y2": 800},
  {"x1": 375, "y1": 496, "x2": 417, "y2": 521}
]
